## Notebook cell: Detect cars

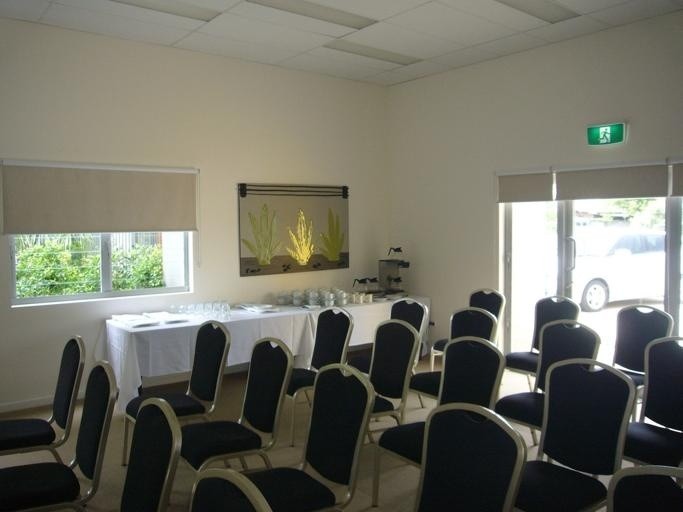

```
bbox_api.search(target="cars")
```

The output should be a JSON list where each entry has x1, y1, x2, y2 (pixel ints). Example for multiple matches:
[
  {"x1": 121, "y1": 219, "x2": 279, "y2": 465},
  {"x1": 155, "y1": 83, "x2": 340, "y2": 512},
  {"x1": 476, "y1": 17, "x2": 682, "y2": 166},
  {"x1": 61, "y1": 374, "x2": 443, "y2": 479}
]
[{"x1": 569, "y1": 229, "x2": 667, "y2": 312}]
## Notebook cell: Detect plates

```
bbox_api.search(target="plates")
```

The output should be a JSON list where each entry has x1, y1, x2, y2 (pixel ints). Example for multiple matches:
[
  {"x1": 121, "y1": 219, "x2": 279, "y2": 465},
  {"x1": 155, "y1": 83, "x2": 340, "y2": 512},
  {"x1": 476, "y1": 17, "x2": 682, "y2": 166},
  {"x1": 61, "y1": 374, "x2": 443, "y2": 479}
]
[{"x1": 238, "y1": 301, "x2": 281, "y2": 314}]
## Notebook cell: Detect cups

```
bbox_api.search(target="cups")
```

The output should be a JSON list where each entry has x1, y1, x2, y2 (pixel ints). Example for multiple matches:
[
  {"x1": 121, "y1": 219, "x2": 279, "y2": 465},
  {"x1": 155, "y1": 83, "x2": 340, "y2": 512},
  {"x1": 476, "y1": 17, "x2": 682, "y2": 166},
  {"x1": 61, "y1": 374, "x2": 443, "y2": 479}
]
[
  {"x1": 166, "y1": 302, "x2": 232, "y2": 321},
  {"x1": 276, "y1": 289, "x2": 374, "y2": 307}
]
